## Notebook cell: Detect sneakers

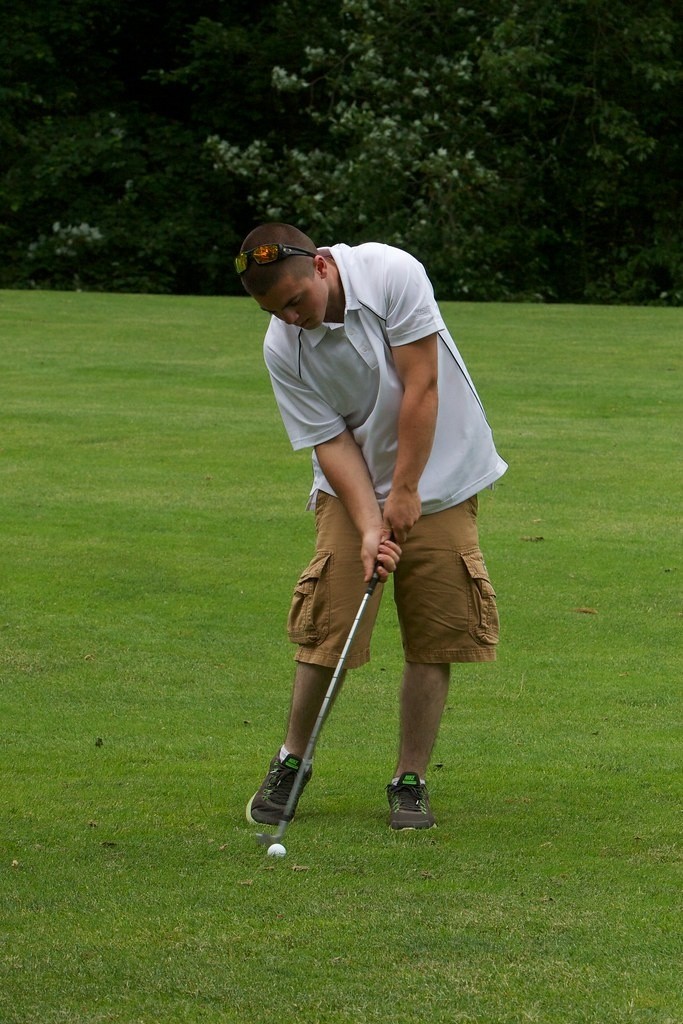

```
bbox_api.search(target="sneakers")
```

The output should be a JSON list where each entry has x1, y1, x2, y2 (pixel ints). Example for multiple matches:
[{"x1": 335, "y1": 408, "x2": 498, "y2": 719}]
[
  {"x1": 245, "y1": 746, "x2": 313, "y2": 828},
  {"x1": 383, "y1": 771, "x2": 437, "y2": 833}
]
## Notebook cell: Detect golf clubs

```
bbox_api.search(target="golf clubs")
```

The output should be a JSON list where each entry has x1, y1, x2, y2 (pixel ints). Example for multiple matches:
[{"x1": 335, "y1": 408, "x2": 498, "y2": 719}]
[{"x1": 257, "y1": 539, "x2": 394, "y2": 846}]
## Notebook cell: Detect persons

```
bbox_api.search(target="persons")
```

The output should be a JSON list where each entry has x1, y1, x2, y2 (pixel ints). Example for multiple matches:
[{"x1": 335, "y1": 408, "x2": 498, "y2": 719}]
[{"x1": 234, "y1": 222, "x2": 509, "y2": 833}]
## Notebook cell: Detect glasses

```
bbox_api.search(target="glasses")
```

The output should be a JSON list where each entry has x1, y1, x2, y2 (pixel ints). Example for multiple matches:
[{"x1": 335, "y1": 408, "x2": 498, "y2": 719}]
[{"x1": 232, "y1": 242, "x2": 316, "y2": 276}]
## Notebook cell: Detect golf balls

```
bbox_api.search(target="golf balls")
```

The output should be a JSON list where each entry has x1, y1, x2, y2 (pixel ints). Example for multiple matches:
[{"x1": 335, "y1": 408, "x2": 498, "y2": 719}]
[{"x1": 267, "y1": 843, "x2": 287, "y2": 858}]
